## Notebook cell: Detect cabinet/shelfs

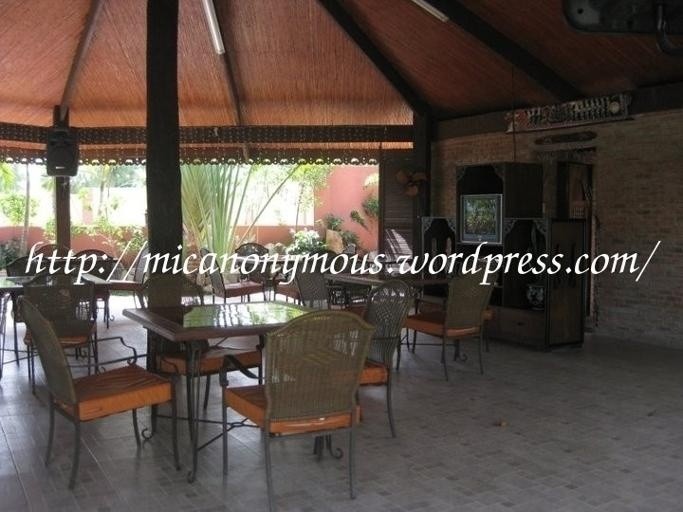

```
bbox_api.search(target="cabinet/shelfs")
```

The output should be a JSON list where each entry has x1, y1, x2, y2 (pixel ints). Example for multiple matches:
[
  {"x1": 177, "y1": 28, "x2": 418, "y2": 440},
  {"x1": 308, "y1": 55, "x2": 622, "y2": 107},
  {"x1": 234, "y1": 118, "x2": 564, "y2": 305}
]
[{"x1": 454, "y1": 164, "x2": 591, "y2": 351}]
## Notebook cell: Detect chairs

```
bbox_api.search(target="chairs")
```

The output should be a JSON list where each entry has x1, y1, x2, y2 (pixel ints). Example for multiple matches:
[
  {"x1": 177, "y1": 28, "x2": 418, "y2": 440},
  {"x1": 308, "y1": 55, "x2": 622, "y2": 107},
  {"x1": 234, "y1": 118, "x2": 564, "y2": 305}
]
[{"x1": 0, "y1": 244, "x2": 499, "y2": 512}]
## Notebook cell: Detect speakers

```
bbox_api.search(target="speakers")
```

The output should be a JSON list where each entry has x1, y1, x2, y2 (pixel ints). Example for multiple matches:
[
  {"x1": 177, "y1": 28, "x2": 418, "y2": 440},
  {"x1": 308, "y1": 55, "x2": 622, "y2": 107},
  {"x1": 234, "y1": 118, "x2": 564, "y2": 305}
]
[{"x1": 45, "y1": 126, "x2": 79, "y2": 176}]
